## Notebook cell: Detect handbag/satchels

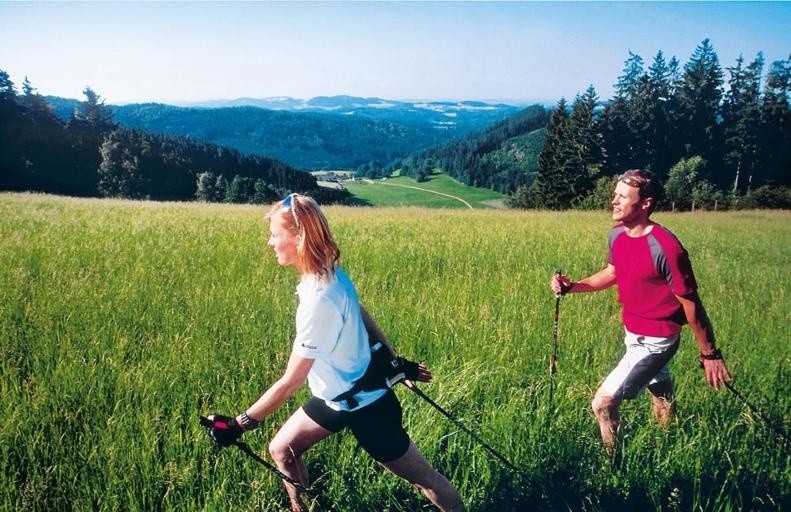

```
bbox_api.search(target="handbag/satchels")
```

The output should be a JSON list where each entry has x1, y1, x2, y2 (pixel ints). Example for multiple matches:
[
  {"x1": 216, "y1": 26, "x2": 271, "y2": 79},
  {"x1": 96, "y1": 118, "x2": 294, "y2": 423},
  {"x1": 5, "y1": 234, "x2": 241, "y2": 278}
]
[{"x1": 355, "y1": 357, "x2": 388, "y2": 392}]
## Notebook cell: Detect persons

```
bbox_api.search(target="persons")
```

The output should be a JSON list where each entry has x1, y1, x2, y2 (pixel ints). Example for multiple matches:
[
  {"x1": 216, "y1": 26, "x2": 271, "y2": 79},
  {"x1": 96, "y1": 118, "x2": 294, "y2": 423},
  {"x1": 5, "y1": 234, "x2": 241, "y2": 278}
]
[
  {"x1": 550, "y1": 167, "x2": 732, "y2": 457},
  {"x1": 208, "y1": 194, "x2": 471, "y2": 512}
]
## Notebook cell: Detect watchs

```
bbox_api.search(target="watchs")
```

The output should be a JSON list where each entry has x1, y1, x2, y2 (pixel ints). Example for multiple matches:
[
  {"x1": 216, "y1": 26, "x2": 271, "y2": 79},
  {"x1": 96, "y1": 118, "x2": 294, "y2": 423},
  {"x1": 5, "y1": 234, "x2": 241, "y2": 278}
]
[
  {"x1": 701, "y1": 348, "x2": 722, "y2": 360},
  {"x1": 240, "y1": 411, "x2": 259, "y2": 431}
]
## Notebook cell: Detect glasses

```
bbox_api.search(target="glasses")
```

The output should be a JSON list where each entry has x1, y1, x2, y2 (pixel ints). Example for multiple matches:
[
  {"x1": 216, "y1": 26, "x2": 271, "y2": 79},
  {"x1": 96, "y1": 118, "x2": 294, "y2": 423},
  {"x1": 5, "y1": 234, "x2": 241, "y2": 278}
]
[
  {"x1": 282, "y1": 193, "x2": 299, "y2": 227},
  {"x1": 617, "y1": 175, "x2": 643, "y2": 188}
]
